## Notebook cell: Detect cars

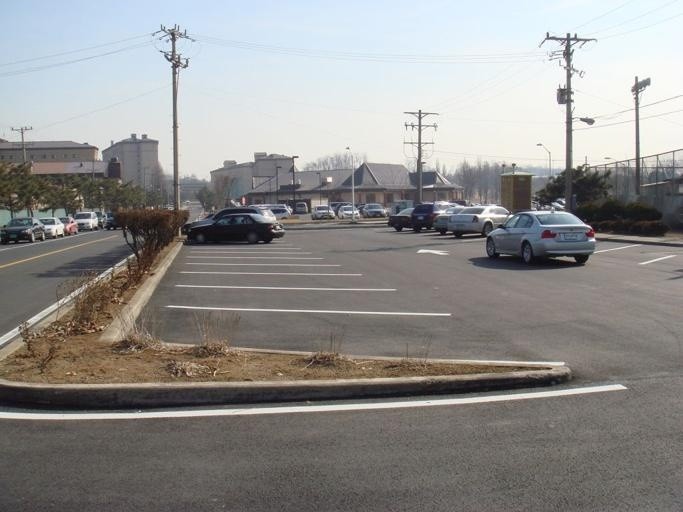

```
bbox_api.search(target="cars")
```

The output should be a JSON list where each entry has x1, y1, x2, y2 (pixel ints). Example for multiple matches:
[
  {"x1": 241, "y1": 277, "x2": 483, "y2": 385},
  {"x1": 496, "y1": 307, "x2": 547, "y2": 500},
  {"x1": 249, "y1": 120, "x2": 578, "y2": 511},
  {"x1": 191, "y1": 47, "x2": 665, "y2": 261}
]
[{"x1": 0, "y1": 197, "x2": 189, "y2": 246}]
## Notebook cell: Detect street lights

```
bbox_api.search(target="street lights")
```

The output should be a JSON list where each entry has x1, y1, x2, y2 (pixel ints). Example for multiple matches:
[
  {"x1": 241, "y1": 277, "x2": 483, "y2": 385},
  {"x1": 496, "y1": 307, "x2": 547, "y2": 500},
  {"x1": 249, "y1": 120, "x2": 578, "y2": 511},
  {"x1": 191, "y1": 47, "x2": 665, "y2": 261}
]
[
  {"x1": 559, "y1": 38, "x2": 596, "y2": 215},
  {"x1": 344, "y1": 145, "x2": 356, "y2": 223},
  {"x1": 630, "y1": 73, "x2": 651, "y2": 198},
  {"x1": 276, "y1": 166, "x2": 281, "y2": 203},
  {"x1": 291, "y1": 154, "x2": 299, "y2": 215},
  {"x1": 536, "y1": 142, "x2": 553, "y2": 177},
  {"x1": 315, "y1": 172, "x2": 321, "y2": 205}
]
